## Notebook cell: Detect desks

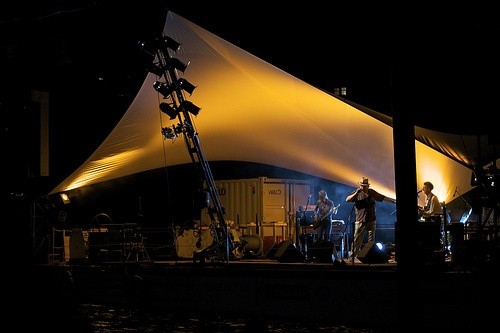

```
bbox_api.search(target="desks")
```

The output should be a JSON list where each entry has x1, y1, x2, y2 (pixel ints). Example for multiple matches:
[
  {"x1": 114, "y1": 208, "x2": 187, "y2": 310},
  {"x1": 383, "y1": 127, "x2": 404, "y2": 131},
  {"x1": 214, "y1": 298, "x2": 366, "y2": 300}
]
[{"x1": 246, "y1": 221, "x2": 287, "y2": 247}]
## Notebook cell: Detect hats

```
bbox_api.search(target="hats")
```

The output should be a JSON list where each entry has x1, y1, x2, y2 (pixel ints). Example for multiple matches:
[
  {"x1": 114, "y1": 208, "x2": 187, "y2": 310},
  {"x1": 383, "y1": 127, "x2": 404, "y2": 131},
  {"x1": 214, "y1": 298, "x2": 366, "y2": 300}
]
[{"x1": 360, "y1": 177, "x2": 369, "y2": 186}]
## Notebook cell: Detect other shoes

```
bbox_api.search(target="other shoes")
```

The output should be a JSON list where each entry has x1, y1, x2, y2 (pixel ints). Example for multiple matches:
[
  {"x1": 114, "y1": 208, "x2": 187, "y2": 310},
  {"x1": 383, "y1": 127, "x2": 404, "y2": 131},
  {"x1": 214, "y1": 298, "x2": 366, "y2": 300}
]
[{"x1": 348, "y1": 257, "x2": 353, "y2": 261}]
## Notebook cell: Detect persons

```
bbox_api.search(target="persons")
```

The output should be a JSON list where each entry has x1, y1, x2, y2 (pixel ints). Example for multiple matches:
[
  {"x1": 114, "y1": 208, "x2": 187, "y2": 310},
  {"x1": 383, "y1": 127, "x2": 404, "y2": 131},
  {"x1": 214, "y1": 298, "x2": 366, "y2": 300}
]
[
  {"x1": 419, "y1": 183, "x2": 443, "y2": 252},
  {"x1": 314, "y1": 190, "x2": 337, "y2": 241},
  {"x1": 345, "y1": 177, "x2": 396, "y2": 261}
]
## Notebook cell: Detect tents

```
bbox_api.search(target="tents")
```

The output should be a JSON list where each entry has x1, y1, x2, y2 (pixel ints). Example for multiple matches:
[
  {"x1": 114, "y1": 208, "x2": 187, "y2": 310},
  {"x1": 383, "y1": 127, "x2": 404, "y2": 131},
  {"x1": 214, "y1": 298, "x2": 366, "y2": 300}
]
[{"x1": 47, "y1": 11, "x2": 491, "y2": 210}]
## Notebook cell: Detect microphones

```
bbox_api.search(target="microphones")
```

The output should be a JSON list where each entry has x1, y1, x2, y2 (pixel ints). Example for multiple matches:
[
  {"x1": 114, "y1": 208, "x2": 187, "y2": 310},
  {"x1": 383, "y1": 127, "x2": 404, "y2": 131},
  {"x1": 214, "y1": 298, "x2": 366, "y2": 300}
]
[{"x1": 453, "y1": 187, "x2": 457, "y2": 197}]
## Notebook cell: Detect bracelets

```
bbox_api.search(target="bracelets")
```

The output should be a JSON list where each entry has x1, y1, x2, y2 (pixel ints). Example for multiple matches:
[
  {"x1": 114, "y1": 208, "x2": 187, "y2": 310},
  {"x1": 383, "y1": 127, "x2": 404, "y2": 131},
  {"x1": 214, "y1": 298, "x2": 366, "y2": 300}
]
[{"x1": 355, "y1": 192, "x2": 359, "y2": 195}]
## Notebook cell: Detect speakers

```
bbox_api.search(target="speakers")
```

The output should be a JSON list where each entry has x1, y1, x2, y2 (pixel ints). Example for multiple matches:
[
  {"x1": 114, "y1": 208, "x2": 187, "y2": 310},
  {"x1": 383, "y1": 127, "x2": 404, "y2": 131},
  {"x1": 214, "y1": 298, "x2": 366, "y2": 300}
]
[{"x1": 266, "y1": 242, "x2": 306, "y2": 263}]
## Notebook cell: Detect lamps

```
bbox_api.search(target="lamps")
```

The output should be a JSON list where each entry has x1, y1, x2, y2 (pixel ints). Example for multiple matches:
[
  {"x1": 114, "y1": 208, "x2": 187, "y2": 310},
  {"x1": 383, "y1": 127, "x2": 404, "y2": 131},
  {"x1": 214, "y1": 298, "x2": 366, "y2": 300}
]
[{"x1": 133, "y1": 36, "x2": 202, "y2": 121}]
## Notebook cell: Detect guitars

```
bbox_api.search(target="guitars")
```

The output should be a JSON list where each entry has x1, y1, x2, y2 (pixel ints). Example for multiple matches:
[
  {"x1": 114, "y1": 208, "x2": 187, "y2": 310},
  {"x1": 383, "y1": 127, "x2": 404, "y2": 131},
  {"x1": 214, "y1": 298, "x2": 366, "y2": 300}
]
[
  {"x1": 312, "y1": 204, "x2": 341, "y2": 230},
  {"x1": 418, "y1": 206, "x2": 431, "y2": 219}
]
